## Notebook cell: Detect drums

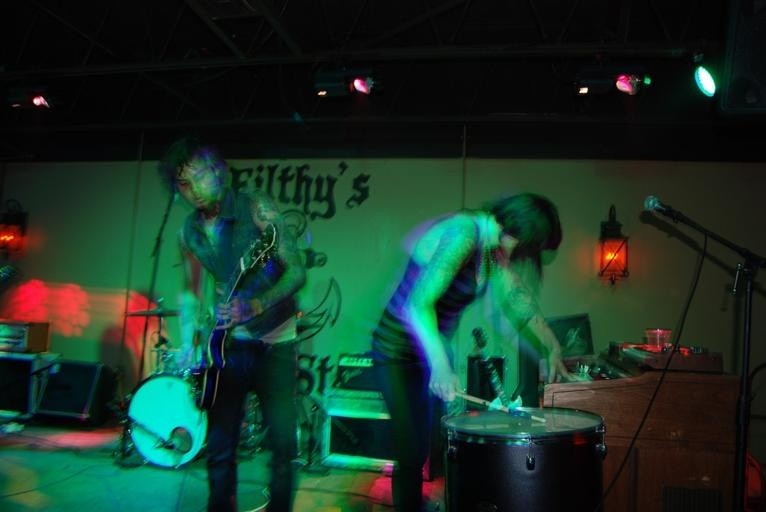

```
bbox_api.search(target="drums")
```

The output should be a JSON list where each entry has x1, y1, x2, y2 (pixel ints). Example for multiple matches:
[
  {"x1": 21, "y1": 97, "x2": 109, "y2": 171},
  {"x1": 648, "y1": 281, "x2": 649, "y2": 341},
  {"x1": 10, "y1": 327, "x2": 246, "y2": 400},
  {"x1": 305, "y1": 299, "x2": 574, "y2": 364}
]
[
  {"x1": 441, "y1": 404, "x2": 605, "y2": 512},
  {"x1": 125, "y1": 374, "x2": 209, "y2": 468}
]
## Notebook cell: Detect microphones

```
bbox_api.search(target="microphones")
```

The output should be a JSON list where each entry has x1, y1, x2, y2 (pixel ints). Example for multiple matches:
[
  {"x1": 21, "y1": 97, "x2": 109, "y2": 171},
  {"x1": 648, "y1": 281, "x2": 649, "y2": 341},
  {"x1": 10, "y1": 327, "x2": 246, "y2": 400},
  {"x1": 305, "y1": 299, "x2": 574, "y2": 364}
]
[{"x1": 644, "y1": 195, "x2": 690, "y2": 225}]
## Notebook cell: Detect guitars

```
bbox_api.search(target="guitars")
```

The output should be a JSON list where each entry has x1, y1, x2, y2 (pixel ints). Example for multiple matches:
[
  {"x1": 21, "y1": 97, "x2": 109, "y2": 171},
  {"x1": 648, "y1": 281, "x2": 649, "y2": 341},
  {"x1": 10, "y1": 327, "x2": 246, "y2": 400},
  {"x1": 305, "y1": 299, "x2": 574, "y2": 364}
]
[{"x1": 190, "y1": 223, "x2": 280, "y2": 408}]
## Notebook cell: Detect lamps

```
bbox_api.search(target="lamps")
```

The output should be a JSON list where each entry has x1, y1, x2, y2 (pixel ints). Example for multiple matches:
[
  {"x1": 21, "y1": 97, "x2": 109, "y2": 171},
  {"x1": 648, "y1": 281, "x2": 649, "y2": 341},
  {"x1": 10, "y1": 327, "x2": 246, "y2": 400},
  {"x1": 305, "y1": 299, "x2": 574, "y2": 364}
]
[
  {"x1": 597, "y1": 203, "x2": 631, "y2": 283},
  {"x1": 0, "y1": 199, "x2": 29, "y2": 253}
]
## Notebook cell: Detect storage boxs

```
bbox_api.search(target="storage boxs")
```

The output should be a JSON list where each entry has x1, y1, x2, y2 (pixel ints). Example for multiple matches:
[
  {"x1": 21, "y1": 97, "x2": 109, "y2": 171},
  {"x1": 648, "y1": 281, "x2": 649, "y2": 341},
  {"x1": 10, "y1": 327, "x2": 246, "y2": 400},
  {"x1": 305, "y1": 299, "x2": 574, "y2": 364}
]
[
  {"x1": 0, "y1": 349, "x2": 59, "y2": 420},
  {"x1": 323, "y1": 386, "x2": 442, "y2": 480}
]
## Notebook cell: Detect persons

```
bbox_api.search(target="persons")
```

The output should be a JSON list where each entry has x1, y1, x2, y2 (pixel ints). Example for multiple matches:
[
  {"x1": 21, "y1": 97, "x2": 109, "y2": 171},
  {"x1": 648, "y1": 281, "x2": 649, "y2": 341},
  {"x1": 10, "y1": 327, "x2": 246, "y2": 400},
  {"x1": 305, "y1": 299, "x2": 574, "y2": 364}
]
[
  {"x1": 161, "y1": 120, "x2": 307, "y2": 512},
  {"x1": 371, "y1": 194, "x2": 572, "y2": 511}
]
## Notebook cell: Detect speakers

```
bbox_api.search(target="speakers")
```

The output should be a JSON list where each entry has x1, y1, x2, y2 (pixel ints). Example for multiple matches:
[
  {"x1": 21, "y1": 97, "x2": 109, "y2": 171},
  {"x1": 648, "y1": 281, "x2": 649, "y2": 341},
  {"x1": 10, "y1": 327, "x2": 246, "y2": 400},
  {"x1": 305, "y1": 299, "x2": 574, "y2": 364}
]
[{"x1": 33, "y1": 359, "x2": 118, "y2": 425}]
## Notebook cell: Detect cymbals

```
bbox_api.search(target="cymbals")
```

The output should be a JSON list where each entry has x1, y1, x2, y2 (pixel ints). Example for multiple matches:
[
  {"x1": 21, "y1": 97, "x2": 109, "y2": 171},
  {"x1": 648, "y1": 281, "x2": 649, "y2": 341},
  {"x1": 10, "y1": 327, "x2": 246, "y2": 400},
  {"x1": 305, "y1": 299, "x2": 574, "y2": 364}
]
[{"x1": 123, "y1": 309, "x2": 185, "y2": 318}]
